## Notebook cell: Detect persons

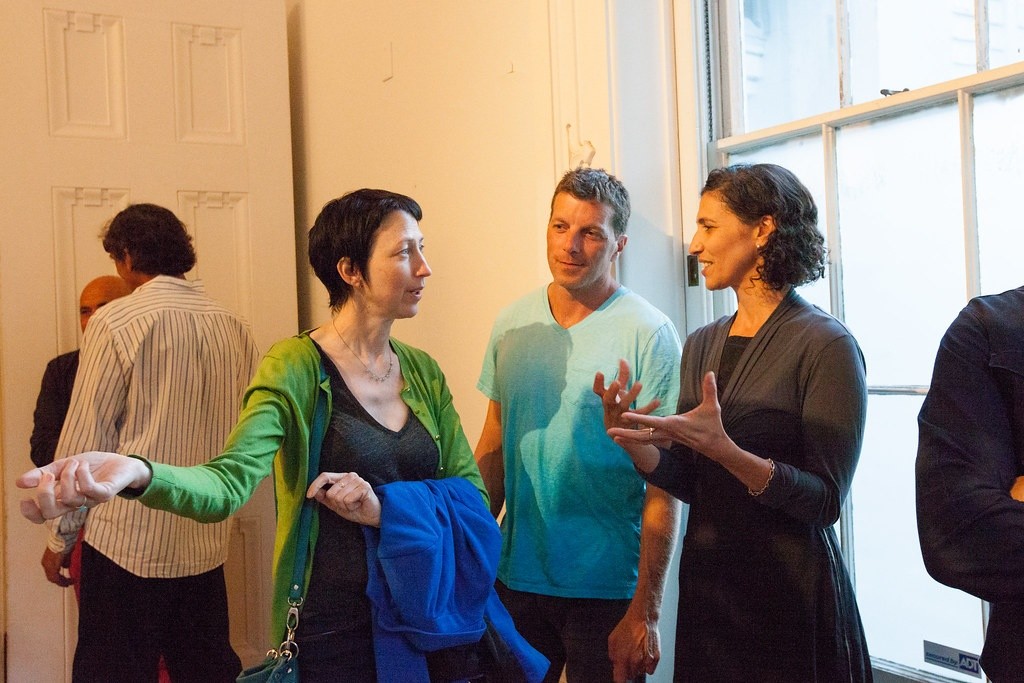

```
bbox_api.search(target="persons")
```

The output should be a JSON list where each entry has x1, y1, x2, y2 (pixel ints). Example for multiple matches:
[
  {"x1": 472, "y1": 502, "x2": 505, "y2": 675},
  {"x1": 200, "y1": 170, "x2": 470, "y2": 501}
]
[
  {"x1": 41, "y1": 202, "x2": 262, "y2": 683},
  {"x1": 16, "y1": 187, "x2": 489, "y2": 683},
  {"x1": 593, "y1": 162, "x2": 874, "y2": 683},
  {"x1": 915, "y1": 284, "x2": 1023, "y2": 683},
  {"x1": 30, "y1": 276, "x2": 170, "y2": 683},
  {"x1": 473, "y1": 168, "x2": 681, "y2": 683}
]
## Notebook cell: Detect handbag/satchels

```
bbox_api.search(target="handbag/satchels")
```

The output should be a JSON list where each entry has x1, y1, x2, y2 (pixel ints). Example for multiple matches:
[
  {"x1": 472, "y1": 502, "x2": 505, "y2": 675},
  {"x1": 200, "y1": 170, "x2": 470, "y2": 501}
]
[{"x1": 236, "y1": 649, "x2": 298, "y2": 683}]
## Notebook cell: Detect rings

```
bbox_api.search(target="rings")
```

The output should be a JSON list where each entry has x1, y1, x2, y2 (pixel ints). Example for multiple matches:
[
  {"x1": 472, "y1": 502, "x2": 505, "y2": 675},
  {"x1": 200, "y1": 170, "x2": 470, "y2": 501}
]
[
  {"x1": 337, "y1": 481, "x2": 345, "y2": 488},
  {"x1": 75, "y1": 496, "x2": 86, "y2": 512},
  {"x1": 649, "y1": 428, "x2": 653, "y2": 438}
]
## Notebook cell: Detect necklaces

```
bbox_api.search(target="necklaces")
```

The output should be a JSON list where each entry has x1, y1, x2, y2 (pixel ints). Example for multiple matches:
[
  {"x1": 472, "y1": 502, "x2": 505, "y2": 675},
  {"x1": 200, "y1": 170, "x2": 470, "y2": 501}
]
[{"x1": 332, "y1": 319, "x2": 392, "y2": 380}]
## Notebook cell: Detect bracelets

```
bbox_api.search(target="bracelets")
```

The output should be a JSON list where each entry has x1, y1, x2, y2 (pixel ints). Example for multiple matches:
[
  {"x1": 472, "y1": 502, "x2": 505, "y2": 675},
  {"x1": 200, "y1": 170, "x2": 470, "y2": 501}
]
[{"x1": 748, "y1": 459, "x2": 774, "y2": 496}]
